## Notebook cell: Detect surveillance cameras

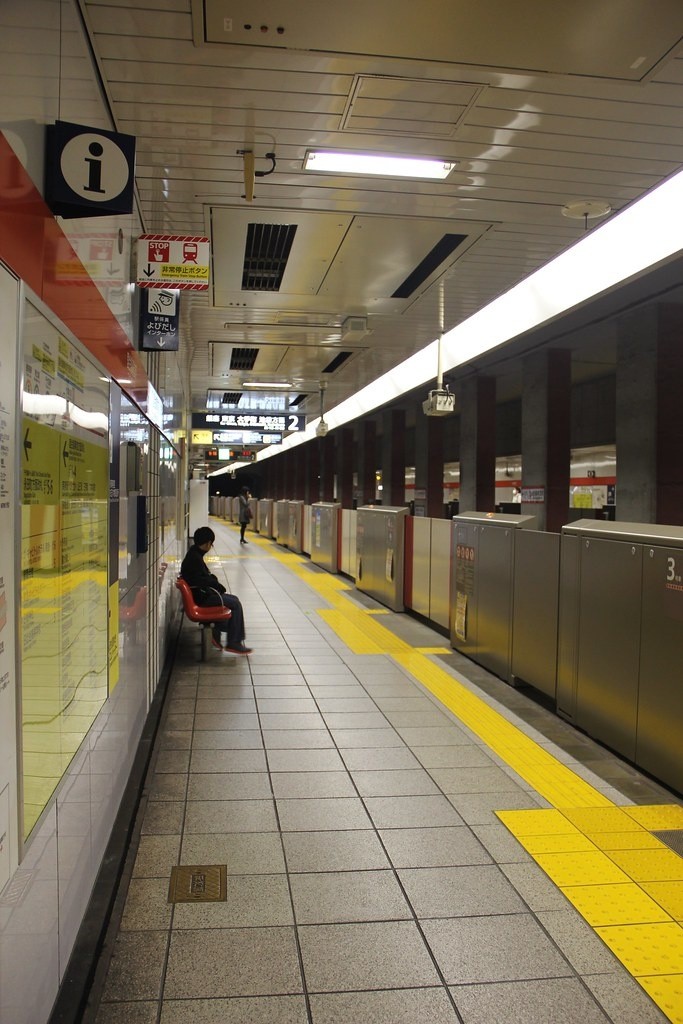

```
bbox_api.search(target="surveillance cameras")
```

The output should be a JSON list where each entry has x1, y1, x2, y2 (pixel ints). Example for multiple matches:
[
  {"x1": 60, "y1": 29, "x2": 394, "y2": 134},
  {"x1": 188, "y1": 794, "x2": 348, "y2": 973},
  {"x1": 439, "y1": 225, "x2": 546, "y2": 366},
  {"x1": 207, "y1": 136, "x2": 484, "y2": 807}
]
[
  {"x1": 316, "y1": 424, "x2": 328, "y2": 437},
  {"x1": 231, "y1": 475, "x2": 236, "y2": 479},
  {"x1": 422, "y1": 394, "x2": 454, "y2": 416}
]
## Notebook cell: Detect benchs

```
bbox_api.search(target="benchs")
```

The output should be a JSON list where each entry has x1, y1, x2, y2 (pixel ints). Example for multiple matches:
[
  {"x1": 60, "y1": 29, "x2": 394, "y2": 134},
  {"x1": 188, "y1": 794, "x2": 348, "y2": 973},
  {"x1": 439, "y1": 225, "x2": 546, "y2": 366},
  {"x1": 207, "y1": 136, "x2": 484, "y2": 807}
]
[{"x1": 179, "y1": 577, "x2": 230, "y2": 660}]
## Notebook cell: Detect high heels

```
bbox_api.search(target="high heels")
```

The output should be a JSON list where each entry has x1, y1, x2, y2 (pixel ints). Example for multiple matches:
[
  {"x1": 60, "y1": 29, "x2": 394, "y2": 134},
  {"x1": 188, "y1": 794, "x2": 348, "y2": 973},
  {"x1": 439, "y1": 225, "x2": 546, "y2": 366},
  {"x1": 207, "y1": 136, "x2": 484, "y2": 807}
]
[{"x1": 240, "y1": 539, "x2": 248, "y2": 544}]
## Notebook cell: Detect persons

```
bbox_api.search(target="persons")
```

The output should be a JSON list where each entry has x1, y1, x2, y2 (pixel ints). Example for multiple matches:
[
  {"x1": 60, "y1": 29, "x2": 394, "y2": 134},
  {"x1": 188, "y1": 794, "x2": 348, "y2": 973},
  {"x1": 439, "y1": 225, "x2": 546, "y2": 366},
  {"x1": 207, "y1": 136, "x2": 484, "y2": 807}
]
[
  {"x1": 239, "y1": 487, "x2": 252, "y2": 543},
  {"x1": 181, "y1": 527, "x2": 252, "y2": 654},
  {"x1": 512, "y1": 487, "x2": 521, "y2": 503}
]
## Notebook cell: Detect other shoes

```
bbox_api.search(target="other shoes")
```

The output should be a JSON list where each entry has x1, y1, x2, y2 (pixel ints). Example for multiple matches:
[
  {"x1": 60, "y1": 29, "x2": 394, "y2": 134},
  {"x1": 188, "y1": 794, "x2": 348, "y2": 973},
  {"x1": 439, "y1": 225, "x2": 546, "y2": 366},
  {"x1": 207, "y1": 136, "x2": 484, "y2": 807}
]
[
  {"x1": 210, "y1": 628, "x2": 223, "y2": 650},
  {"x1": 226, "y1": 642, "x2": 252, "y2": 654}
]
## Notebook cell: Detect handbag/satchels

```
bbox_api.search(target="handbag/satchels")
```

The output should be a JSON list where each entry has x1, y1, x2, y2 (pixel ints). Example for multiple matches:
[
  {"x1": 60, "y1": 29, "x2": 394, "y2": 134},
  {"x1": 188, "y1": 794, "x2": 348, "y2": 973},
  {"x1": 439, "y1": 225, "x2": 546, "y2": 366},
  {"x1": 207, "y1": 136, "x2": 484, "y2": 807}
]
[{"x1": 246, "y1": 508, "x2": 254, "y2": 519}]
